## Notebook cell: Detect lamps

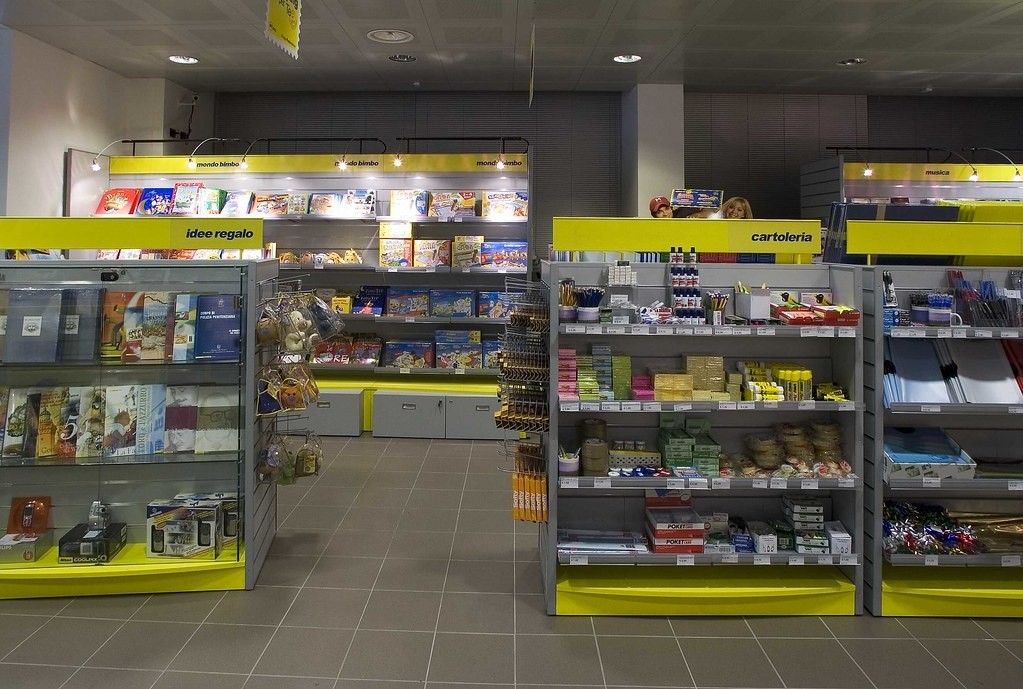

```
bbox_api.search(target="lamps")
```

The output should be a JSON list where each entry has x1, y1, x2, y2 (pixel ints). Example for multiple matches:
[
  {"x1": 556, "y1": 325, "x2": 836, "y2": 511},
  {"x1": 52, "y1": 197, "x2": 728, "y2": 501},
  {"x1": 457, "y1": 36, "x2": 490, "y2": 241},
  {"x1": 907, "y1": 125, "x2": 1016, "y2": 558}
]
[
  {"x1": 240, "y1": 138, "x2": 263, "y2": 168},
  {"x1": 189, "y1": 138, "x2": 217, "y2": 168},
  {"x1": 844, "y1": 145, "x2": 871, "y2": 176},
  {"x1": 497, "y1": 136, "x2": 504, "y2": 169},
  {"x1": 340, "y1": 137, "x2": 358, "y2": 170},
  {"x1": 971, "y1": 147, "x2": 1022, "y2": 181},
  {"x1": 92, "y1": 139, "x2": 132, "y2": 172},
  {"x1": 939, "y1": 146, "x2": 978, "y2": 181},
  {"x1": 394, "y1": 136, "x2": 411, "y2": 166}
]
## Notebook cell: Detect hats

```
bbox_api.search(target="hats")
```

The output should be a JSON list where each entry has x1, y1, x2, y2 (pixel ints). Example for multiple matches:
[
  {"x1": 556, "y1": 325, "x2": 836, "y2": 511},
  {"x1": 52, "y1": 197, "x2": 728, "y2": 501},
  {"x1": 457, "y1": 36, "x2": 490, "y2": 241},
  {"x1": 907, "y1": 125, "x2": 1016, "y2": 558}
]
[{"x1": 650, "y1": 196, "x2": 670, "y2": 213}]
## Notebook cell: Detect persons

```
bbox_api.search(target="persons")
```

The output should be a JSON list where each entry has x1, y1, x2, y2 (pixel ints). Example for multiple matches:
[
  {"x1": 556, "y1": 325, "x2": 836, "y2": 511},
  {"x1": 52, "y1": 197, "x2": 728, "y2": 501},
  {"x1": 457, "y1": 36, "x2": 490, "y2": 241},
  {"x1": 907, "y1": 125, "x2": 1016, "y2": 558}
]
[
  {"x1": 104, "y1": 411, "x2": 131, "y2": 447},
  {"x1": 197, "y1": 393, "x2": 238, "y2": 451},
  {"x1": 722, "y1": 197, "x2": 753, "y2": 219},
  {"x1": 169, "y1": 386, "x2": 197, "y2": 407},
  {"x1": 164, "y1": 430, "x2": 196, "y2": 451},
  {"x1": 649, "y1": 196, "x2": 717, "y2": 218}
]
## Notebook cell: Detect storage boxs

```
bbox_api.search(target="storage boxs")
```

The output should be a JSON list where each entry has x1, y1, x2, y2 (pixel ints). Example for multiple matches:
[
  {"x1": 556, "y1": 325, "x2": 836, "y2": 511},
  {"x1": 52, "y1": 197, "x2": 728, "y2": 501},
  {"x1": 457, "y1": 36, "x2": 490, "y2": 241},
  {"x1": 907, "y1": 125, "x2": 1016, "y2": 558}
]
[
  {"x1": 771, "y1": 302, "x2": 860, "y2": 327},
  {"x1": 884, "y1": 427, "x2": 976, "y2": 485},
  {"x1": 0, "y1": 529, "x2": 53, "y2": 564},
  {"x1": 145, "y1": 490, "x2": 237, "y2": 558},
  {"x1": 660, "y1": 413, "x2": 722, "y2": 478},
  {"x1": 733, "y1": 286, "x2": 772, "y2": 319},
  {"x1": 97, "y1": 184, "x2": 533, "y2": 373},
  {"x1": 60, "y1": 521, "x2": 127, "y2": 563},
  {"x1": 643, "y1": 485, "x2": 853, "y2": 554}
]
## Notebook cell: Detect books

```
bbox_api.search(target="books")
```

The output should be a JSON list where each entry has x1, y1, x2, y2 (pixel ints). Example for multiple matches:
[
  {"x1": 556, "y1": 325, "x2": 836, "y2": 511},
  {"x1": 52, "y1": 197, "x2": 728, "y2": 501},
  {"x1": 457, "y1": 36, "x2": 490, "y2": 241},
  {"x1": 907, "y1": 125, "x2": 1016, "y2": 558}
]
[
  {"x1": 0, "y1": 284, "x2": 246, "y2": 457},
  {"x1": 96, "y1": 242, "x2": 277, "y2": 260}
]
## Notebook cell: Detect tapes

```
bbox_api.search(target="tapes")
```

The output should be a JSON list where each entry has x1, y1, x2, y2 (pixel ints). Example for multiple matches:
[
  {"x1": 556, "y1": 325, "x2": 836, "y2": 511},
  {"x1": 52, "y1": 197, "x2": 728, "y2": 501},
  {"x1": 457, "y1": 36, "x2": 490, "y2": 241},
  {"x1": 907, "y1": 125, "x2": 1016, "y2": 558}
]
[{"x1": 582, "y1": 418, "x2": 609, "y2": 476}]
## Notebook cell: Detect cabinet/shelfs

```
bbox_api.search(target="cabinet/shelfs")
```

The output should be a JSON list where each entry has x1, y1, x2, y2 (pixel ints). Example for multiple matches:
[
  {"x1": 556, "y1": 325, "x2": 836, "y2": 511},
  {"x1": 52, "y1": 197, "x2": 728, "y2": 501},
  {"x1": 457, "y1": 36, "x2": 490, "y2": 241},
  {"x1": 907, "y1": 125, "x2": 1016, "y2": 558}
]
[
  {"x1": 550, "y1": 262, "x2": 865, "y2": 616},
  {"x1": 0, "y1": 260, "x2": 254, "y2": 599},
  {"x1": 876, "y1": 266, "x2": 1023, "y2": 617},
  {"x1": 255, "y1": 209, "x2": 528, "y2": 441}
]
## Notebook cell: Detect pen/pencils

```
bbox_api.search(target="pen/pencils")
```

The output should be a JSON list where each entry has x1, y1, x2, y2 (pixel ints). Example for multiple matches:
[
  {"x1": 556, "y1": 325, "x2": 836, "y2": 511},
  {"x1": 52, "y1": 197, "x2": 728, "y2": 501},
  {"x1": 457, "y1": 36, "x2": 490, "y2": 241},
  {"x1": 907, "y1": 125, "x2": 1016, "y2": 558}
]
[
  {"x1": 706, "y1": 290, "x2": 730, "y2": 311},
  {"x1": 561, "y1": 283, "x2": 605, "y2": 307},
  {"x1": 883, "y1": 270, "x2": 893, "y2": 302},
  {"x1": 558, "y1": 444, "x2": 581, "y2": 460},
  {"x1": 909, "y1": 270, "x2": 1012, "y2": 327}
]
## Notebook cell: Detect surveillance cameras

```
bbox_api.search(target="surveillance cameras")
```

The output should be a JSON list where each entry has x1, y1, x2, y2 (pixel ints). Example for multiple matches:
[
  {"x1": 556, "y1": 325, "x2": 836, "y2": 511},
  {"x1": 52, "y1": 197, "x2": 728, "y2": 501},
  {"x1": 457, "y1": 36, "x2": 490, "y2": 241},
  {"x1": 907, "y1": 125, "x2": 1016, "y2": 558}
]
[{"x1": 192, "y1": 95, "x2": 199, "y2": 101}]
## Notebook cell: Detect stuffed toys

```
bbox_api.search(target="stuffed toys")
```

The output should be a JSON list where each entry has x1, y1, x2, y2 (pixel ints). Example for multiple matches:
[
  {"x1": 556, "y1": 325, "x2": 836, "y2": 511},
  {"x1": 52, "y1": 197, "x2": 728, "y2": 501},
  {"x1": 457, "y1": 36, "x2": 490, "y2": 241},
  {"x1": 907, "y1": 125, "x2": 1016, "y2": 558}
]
[
  {"x1": 257, "y1": 443, "x2": 296, "y2": 481},
  {"x1": 258, "y1": 366, "x2": 319, "y2": 414},
  {"x1": 284, "y1": 302, "x2": 344, "y2": 352}
]
